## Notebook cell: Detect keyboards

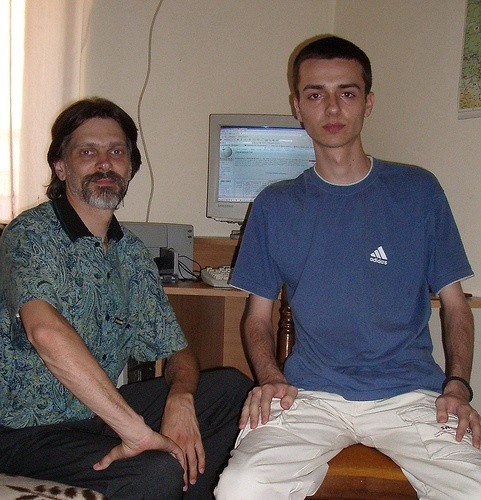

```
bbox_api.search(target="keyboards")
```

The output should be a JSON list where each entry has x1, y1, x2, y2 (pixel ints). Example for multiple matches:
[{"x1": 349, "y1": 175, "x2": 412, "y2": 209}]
[{"x1": 201, "y1": 267, "x2": 237, "y2": 288}]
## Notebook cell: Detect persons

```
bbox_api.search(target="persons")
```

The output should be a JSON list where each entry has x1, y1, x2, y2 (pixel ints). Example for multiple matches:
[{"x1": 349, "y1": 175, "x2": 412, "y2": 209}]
[
  {"x1": 0, "y1": 96, "x2": 258, "y2": 499},
  {"x1": 213, "y1": 35, "x2": 481, "y2": 500},
  {"x1": 441, "y1": 376, "x2": 474, "y2": 403}
]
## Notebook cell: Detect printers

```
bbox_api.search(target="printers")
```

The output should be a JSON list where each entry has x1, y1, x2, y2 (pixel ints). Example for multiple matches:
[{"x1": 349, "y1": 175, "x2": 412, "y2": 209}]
[{"x1": 118, "y1": 222, "x2": 196, "y2": 278}]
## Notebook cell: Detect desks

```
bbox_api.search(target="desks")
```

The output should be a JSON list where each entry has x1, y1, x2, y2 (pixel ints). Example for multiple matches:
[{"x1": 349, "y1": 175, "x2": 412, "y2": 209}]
[{"x1": 154, "y1": 236, "x2": 282, "y2": 387}]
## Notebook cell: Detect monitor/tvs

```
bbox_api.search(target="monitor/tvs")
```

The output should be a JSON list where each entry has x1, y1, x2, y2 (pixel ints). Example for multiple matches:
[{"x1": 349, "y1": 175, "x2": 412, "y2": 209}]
[{"x1": 206, "y1": 114, "x2": 317, "y2": 222}]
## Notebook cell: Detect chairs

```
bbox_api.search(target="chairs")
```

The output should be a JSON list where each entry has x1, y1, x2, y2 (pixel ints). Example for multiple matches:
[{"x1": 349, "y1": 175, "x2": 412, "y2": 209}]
[{"x1": 276, "y1": 282, "x2": 420, "y2": 500}]
